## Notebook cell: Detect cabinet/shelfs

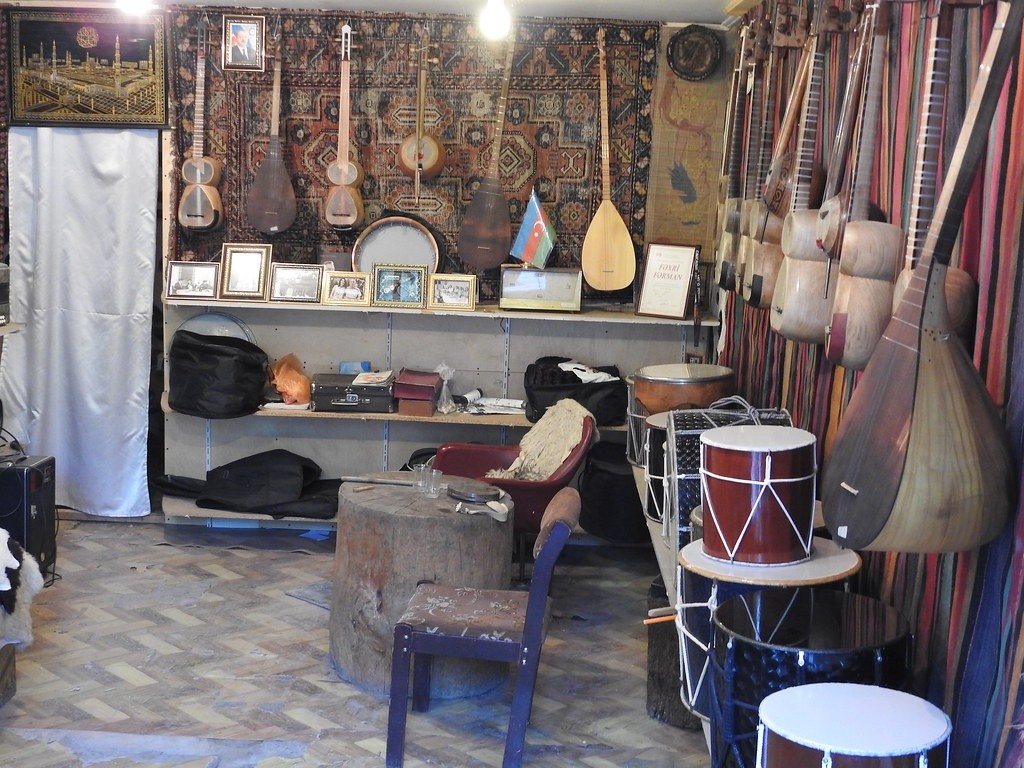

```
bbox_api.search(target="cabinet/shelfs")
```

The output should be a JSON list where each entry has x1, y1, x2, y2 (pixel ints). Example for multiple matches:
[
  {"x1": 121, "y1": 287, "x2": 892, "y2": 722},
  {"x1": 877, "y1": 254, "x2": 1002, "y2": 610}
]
[{"x1": 160, "y1": 291, "x2": 721, "y2": 551}]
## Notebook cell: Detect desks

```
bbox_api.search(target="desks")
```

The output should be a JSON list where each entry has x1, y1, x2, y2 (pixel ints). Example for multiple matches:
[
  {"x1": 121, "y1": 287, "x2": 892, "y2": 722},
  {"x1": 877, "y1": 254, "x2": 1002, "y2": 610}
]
[{"x1": 331, "y1": 473, "x2": 514, "y2": 698}]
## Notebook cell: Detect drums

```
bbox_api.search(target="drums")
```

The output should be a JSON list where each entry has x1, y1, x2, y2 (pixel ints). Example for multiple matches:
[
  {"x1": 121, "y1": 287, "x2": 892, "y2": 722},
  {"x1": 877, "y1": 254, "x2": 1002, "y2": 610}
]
[
  {"x1": 754, "y1": 680, "x2": 953, "y2": 768},
  {"x1": 632, "y1": 362, "x2": 736, "y2": 524},
  {"x1": 676, "y1": 534, "x2": 865, "y2": 723},
  {"x1": 641, "y1": 406, "x2": 817, "y2": 567},
  {"x1": 708, "y1": 589, "x2": 912, "y2": 768}
]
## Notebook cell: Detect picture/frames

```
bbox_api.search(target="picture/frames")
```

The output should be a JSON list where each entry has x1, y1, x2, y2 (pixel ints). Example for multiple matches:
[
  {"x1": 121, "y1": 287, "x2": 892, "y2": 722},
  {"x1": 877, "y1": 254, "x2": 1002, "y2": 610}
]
[
  {"x1": 271, "y1": 262, "x2": 323, "y2": 305},
  {"x1": 426, "y1": 274, "x2": 477, "y2": 311},
  {"x1": 371, "y1": 263, "x2": 428, "y2": 309},
  {"x1": 9, "y1": 7, "x2": 169, "y2": 131},
  {"x1": 322, "y1": 271, "x2": 371, "y2": 308},
  {"x1": 221, "y1": 242, "x2": 272, "y2": 301},
  {"x1": 635, "y1": 241, "x2": 701, "y2": 321},
  {"x1": 165, "y1": 260, "x2": 220, "y2": 301},
  {"x1": 221, "y1": 14, "x2": 266, "y2": 73}
]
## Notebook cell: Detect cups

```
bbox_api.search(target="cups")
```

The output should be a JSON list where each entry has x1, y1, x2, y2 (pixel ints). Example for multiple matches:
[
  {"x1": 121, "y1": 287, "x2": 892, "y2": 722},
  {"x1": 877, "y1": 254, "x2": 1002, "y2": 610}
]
[
  {"x1": 424, "y1": 469, "x2": 443, "y2": 498},
  {"x1": 414, "y1": 464, "x2": 430, "y2": 491}
]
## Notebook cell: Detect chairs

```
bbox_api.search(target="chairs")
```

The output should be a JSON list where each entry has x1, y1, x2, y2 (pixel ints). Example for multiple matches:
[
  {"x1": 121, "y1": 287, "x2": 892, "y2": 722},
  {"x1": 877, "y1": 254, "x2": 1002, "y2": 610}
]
[
  {"x1": 430, "y1": 397, "x2": 598, "y2": 588},
  {"x1": 383, "y1": 488, "x2": 580, "y2": 768}
]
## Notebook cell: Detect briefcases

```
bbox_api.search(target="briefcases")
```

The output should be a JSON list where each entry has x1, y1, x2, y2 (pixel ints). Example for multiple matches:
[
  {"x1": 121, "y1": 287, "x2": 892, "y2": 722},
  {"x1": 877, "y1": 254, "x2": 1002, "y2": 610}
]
[{"x1": 310, "y1": 374, "x2": 396, "y2": 413}]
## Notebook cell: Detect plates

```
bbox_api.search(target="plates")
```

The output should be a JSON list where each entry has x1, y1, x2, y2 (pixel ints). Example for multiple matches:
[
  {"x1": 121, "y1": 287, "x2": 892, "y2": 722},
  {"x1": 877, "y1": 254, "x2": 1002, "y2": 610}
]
[{"x1": 351, "y1": 216, "x2": 438, "y2": 273}]
